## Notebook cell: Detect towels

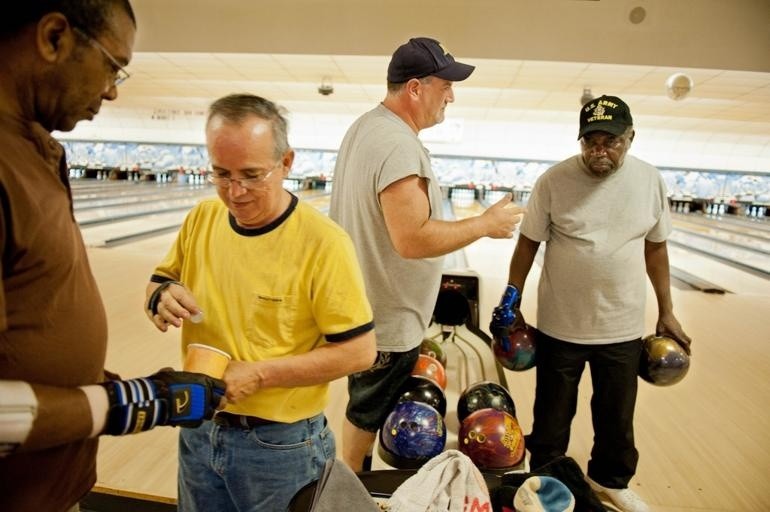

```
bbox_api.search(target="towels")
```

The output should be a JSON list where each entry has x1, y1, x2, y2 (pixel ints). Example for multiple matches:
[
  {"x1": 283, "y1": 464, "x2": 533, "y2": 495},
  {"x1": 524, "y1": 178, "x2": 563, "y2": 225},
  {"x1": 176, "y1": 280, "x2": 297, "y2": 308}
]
[{"x1": 380, "y1": 449, "x2": 493, "y2": 512}]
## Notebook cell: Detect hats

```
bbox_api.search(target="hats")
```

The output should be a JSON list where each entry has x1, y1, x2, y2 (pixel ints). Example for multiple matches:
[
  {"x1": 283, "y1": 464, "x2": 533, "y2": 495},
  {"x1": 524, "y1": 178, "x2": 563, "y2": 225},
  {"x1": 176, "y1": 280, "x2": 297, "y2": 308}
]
[
  {"x1": 387, "y1": 37, "x2": 474, "y2": 82},
  {"x1": 578, "y1": 95, "x2": 632, "y2": 140}
]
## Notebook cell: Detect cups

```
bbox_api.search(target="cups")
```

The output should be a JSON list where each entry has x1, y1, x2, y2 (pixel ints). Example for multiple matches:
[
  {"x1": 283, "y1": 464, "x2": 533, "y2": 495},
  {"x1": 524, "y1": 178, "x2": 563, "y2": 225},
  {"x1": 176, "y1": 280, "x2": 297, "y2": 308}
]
[{"x1": 186, "y1": 343, "x2": 233, "y2": 386}]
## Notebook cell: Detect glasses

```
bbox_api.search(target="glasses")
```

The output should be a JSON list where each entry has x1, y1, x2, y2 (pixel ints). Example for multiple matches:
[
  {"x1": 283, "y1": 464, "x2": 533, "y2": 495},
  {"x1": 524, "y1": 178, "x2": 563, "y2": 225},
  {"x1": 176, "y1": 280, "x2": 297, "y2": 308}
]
[
  {"x1": 207, "y1": 161, "x2": 280, "y2": 186},
  {"x1": 72, "y1": 27, "x2": 129, "y2": 86}
]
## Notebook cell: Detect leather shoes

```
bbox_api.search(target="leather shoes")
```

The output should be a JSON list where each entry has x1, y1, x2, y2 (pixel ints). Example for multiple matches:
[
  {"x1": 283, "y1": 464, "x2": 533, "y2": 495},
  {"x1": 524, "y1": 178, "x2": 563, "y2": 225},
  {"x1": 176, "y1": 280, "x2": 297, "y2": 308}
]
[{"x1": 585, "y1": 474, "x2": 648, "y2": 512}]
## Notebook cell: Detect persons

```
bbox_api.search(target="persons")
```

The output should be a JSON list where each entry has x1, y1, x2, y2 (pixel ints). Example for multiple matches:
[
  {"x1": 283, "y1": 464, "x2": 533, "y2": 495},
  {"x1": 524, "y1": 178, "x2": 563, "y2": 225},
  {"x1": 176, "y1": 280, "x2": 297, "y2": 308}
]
[
  {"x1": 0, "y1": 2, "x2": 230, "y2": 510},
  {"x1": 142, "y1": 91, "x2": 378, "y2": 511},
  {"x1": 333, "y1": 35, "x2": 527, "y2": 473},
  {"x1": 487, "y1": 92, "x2": 691, "y2": 511}
]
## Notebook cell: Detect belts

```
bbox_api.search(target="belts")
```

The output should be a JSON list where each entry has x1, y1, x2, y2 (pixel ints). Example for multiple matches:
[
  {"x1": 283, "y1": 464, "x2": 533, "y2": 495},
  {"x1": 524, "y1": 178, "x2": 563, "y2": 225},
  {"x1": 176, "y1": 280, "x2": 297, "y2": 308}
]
[{"x1": 215, "y1": 411, "x2": 274, "y2": 430}]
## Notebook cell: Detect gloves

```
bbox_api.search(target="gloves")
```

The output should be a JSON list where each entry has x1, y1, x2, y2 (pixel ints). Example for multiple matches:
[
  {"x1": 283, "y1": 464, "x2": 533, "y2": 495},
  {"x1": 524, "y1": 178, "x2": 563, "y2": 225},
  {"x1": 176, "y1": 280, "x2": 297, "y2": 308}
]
[
  {"x1": 147, "y1": 368, "x2": 225, "y2": 428},
  {"x1": 489, "y1": 284, "x2": 528, "y2": 353}
]
